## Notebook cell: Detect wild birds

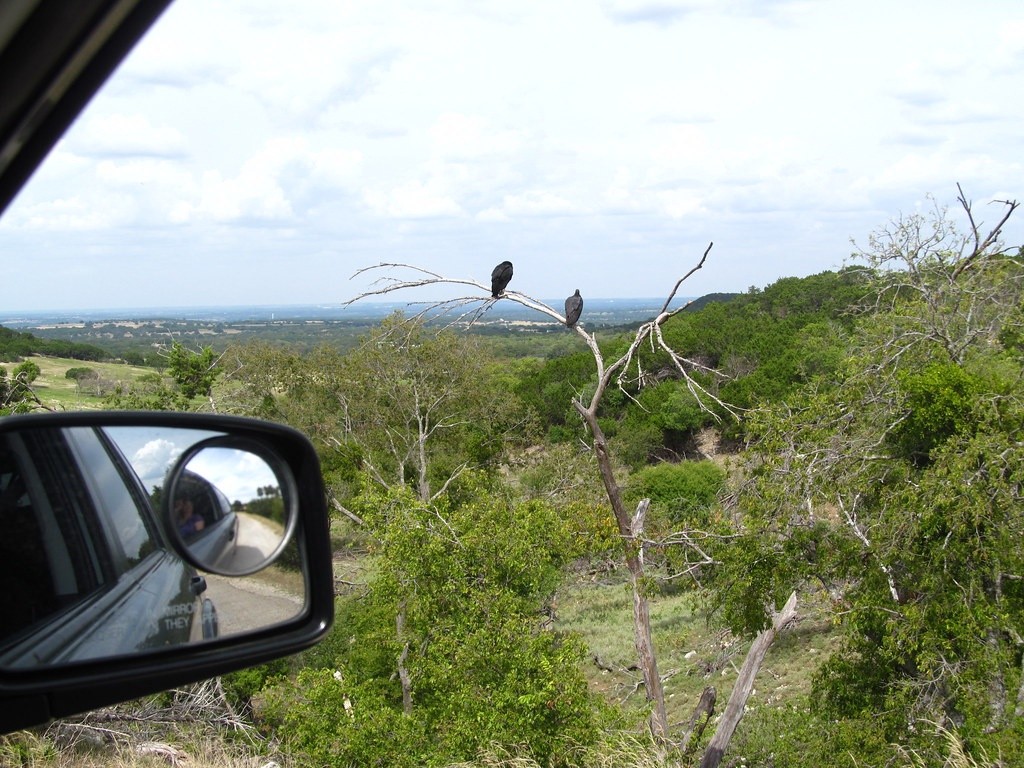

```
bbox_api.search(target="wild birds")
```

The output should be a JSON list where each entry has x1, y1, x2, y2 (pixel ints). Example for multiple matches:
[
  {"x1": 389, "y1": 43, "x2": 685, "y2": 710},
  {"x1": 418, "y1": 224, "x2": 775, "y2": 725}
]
[
  {"x1": 491, "y1": 261, "x2": 513, "y2": 299},
  {"x1": 565, "y1": 289, "x2": 583, "y2": 328}
]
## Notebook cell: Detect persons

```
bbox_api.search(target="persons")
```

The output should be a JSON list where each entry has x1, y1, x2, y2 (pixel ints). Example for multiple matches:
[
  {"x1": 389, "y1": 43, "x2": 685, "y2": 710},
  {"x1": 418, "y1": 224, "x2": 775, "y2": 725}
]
[{"x1": 174, "y1": 500, "x2": 204, "y2": 538}]
